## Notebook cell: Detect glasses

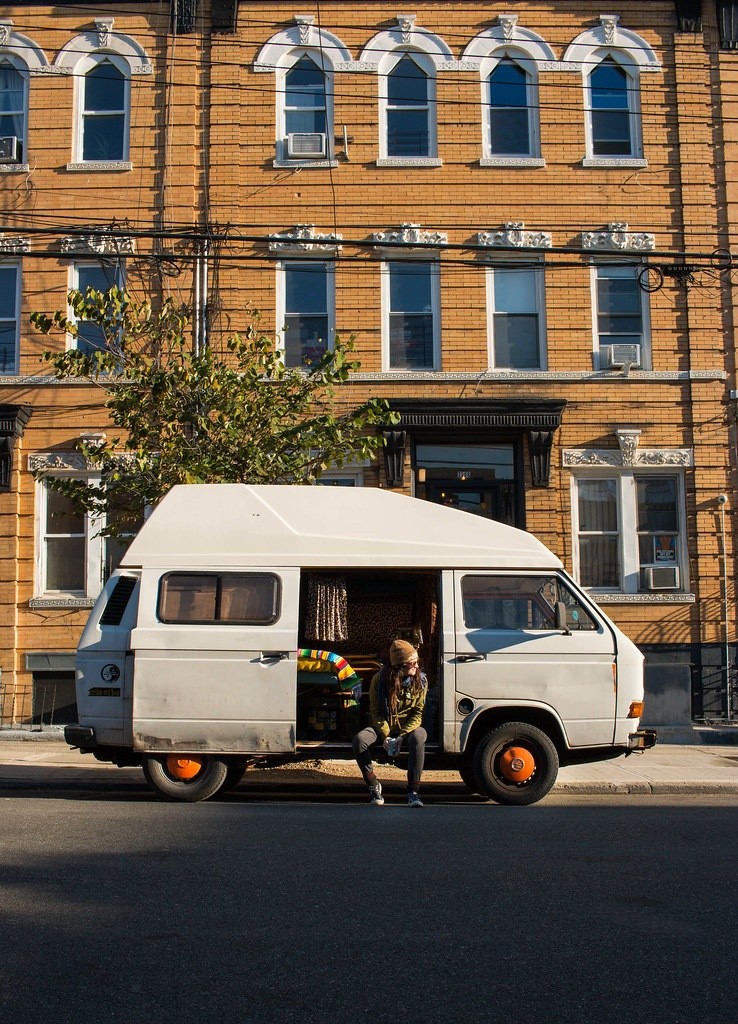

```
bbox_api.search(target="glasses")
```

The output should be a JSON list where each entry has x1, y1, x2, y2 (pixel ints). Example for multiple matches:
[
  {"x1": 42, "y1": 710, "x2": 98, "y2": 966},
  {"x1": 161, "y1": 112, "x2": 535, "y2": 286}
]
[{"x1": 400, "y1": 660, "x2": 419, "y2": 667}]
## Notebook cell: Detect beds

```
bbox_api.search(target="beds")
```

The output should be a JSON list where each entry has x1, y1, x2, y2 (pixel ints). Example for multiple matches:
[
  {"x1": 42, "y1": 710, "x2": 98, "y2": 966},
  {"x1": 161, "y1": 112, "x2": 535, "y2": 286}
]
[{"x1": 298, "y1": 652, "x2": 382, "y2": 737}]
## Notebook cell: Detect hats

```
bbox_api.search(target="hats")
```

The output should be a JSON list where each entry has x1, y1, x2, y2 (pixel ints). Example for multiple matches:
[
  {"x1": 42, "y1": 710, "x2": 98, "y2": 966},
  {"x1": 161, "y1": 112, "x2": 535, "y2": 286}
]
[{"x1": 389, "y1": 639, "x2": 418, "y2": 665}]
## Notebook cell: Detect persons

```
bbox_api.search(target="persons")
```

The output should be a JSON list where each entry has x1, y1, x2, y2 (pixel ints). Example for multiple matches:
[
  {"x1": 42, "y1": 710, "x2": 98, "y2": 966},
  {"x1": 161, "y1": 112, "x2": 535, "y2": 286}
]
[{"x1": 352, "y1": 640, "x2": 428, "y2": 806}]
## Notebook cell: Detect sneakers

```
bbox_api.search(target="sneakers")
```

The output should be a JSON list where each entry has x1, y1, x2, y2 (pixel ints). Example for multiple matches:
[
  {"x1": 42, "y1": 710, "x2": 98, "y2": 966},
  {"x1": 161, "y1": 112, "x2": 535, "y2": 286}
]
[
  {"x1": 367, "y1": 781, "x2": 385, "y2": 805},
  {"x1": 408, "y1": 791, "x2": 423, "y2": 807}
]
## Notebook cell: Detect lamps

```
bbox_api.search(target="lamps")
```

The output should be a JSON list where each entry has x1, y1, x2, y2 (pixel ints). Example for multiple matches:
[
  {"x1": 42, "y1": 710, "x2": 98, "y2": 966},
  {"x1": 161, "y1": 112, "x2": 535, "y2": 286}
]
[{"x1": 417, "y1": 466, "x2": 427, "y2": 482}]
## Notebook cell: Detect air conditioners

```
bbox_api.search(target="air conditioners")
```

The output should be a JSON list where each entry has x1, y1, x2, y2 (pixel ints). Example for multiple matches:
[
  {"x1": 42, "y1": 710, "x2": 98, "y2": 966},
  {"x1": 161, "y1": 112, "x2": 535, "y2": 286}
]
[
  {"x1": 0, "y1": 135, "x2": 23, "y2": 165},
  {"x1": 607, "y1": 344, "x2": 640, "y2": 368},
  {"x1": 644, "y1": 566, "x2": 679, "y2": 591},
  {"x1": 288, "y1": 133, "x2": 328, "y2": 159}
]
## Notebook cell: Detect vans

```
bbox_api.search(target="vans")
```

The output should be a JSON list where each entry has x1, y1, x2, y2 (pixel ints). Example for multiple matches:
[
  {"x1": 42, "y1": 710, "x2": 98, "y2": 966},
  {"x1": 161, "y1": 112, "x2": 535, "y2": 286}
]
[{"x1": 63, "y1": 482, "x2": 660, "y2": 808}]
